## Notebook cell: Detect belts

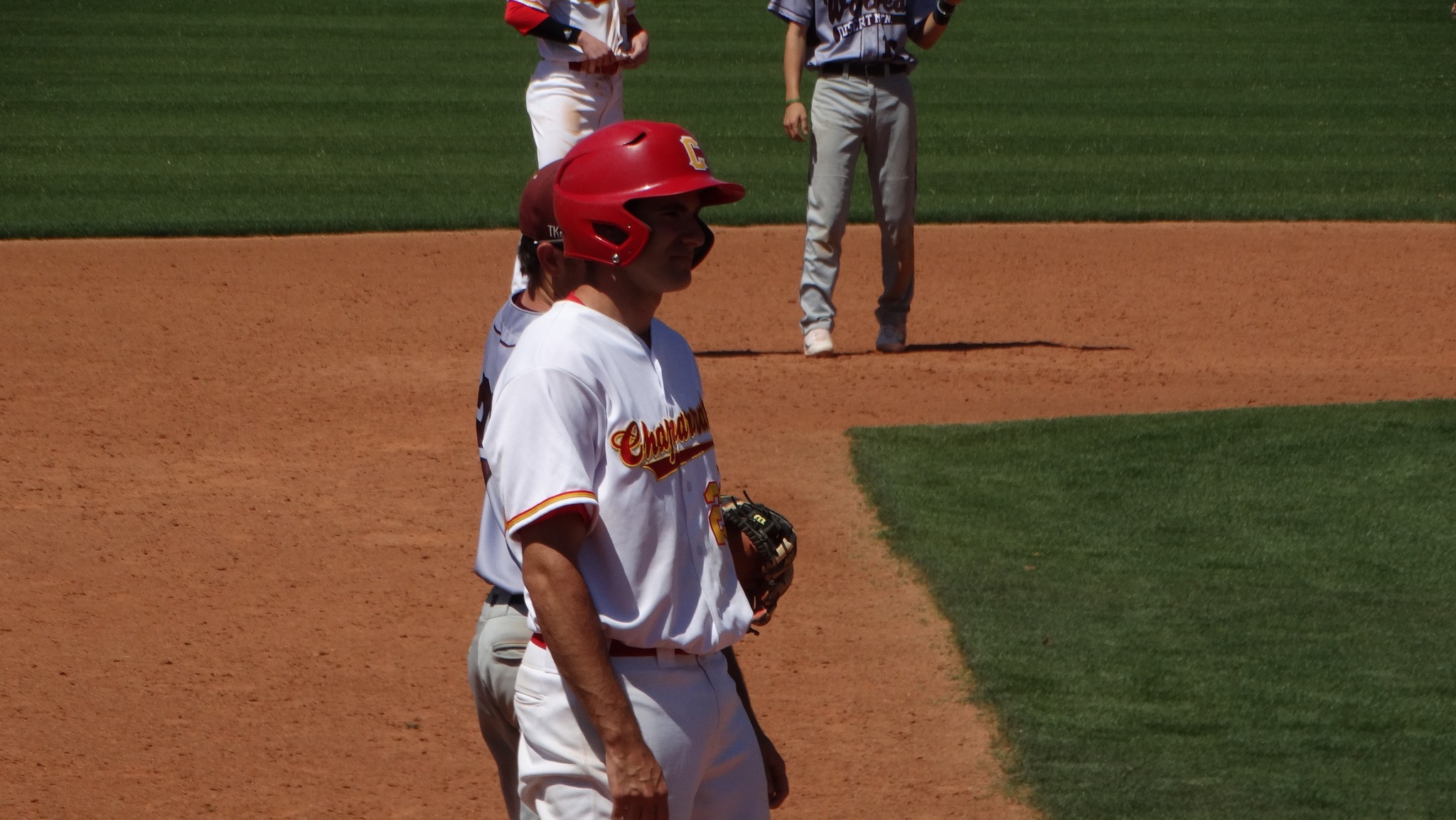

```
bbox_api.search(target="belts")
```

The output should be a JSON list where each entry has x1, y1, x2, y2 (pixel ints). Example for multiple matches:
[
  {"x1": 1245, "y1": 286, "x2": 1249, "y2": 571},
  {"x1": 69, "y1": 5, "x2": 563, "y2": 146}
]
[
  {"x1": 822, "y1": 62, "x2": 908, "y2": 77},
  {"x1": 530, "y1": 632, "x2": 692, "y2": 655},
  {"x1": 569, "y1": 61, "x2": 618, "y2": 75},
  {"x1": 487, "y1": 593, "x2": 525, "y2": 605}
]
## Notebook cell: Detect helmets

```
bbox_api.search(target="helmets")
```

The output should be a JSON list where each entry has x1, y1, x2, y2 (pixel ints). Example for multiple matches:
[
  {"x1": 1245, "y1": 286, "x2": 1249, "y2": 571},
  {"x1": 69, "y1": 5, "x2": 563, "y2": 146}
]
[
  {"x1": 552, "y1": 122, "x2": 745, "y2": 265},
  {"x1": 518, "y1": 158, "x2": 565, "y2": 240}
]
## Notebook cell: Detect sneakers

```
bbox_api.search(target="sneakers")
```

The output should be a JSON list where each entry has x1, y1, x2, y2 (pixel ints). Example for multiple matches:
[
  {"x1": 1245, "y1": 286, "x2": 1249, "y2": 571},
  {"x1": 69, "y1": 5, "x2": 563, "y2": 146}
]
[
  {"x1": 802, "y1": 328, "x2": 834, "y2": 356},
  {"x1": 876, "y1": 322, "x2": 908, "y2": 352}
]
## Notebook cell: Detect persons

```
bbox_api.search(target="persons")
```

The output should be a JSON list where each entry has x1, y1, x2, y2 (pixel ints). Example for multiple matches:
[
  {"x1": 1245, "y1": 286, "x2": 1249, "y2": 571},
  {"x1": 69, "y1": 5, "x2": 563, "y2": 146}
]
[
  {"x1": 766, "y1": 0, "x2": 962, "y2": 358},
  {"x1": 470, "y1": 0, "x2": 797, "y2": 820}
]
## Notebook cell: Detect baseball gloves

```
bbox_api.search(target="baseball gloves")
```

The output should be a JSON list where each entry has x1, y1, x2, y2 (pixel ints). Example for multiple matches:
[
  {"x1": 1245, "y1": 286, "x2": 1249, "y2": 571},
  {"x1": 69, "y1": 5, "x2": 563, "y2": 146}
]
[{"x1": 720, "y1": 495, "x2": 798, "y2": 627}]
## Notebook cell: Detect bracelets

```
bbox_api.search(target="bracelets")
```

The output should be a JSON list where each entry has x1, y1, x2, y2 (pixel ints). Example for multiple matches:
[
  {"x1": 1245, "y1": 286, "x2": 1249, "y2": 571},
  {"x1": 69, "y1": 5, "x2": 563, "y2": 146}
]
[
  {"x1": 785, "y1": 98, "x2": 800, "y2": 107},
  {"x1": 932, "y1": 0, "x2": 956, "y2": 25}
]
